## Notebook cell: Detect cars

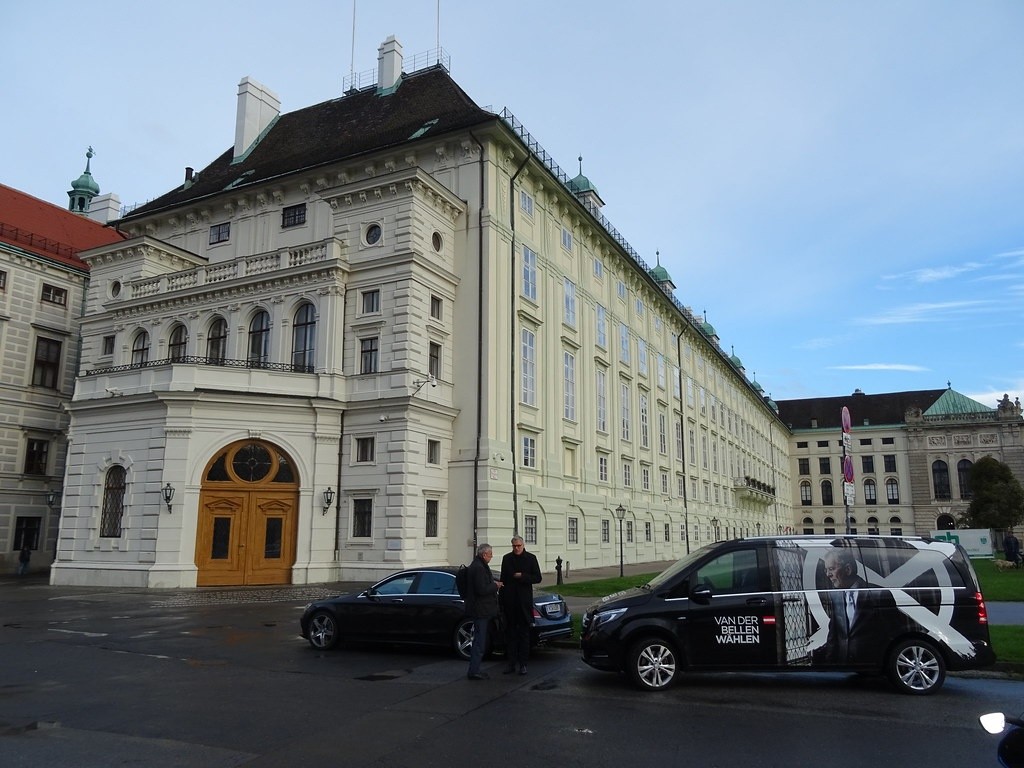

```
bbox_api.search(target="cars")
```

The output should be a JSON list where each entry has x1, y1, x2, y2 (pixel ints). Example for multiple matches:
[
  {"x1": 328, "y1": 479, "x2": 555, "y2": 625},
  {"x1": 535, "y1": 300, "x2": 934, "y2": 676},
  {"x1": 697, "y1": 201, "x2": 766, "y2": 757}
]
[{"x1": 301, "y1": 566, "x2": 575, "y2": 661}]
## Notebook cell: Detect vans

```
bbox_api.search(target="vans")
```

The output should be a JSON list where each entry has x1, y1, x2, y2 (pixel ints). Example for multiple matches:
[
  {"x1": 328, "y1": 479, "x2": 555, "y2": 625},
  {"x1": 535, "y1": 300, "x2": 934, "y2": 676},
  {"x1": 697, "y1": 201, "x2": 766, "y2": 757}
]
[{"x1": 581, "y1": 535, "x2": 993, "y2": 696}]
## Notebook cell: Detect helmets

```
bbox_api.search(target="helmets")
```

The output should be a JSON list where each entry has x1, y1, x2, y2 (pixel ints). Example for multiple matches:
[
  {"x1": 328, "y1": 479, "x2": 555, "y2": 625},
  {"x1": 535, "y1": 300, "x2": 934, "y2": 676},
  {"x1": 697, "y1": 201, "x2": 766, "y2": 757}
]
[{"x1": 997, "y1": 728, "x2": 1024, "y2": 768}]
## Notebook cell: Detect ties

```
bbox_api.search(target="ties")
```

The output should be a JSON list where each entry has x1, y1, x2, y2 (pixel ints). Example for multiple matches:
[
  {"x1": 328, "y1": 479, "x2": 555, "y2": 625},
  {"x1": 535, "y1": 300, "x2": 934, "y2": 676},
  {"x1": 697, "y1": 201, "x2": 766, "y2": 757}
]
[{"x1": 848, "y1": 590, "x2": 856, "y2": 628}]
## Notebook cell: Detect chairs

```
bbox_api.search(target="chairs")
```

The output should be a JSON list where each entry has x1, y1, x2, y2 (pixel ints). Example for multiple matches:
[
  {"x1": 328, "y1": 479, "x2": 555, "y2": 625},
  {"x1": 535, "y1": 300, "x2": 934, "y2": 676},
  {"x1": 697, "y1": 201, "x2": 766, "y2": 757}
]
[{"x1": 741, "y1": 566, "x2": 760, "y2": 592}]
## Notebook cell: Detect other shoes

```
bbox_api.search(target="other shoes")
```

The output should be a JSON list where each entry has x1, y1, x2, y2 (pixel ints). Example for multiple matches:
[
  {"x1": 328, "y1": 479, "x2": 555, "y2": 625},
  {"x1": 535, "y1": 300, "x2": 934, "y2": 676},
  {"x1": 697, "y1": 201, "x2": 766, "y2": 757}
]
[
  {"x1": 467, "y1": 672, "x2": 489, "y2": 680},
  {"x1": 503, "y1": 666, "x2": 516, "y2": 674},
  {"x1": 520, "y1": 665, "x2": 529, "y2": 674}
]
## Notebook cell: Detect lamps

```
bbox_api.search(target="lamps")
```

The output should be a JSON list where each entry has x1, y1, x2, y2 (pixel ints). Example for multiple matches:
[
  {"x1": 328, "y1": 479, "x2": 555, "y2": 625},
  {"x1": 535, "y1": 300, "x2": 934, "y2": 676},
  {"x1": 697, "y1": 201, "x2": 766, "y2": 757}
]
[
  {"x1": 45, "y1": 489, "x2": 61, "y2": 516},
  {"x1": 323, "y1": 487, "x2": 335, "y2": 516},
  {"x1": 161, "y1": 482, "x2": 175, "y2": 514}
]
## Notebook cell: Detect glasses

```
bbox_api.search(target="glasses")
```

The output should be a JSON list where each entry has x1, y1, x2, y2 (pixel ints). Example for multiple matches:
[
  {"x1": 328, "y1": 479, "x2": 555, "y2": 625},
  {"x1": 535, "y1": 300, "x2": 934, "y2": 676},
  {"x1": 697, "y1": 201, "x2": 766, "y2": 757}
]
[{"x1": 512, "y1": 544, "x2": 523, "y2": 547}]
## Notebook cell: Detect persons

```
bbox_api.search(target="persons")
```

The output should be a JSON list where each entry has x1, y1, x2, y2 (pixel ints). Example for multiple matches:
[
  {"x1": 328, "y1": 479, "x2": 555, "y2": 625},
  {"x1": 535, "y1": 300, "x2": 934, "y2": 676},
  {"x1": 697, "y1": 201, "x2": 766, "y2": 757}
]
[
  {"x1": 805, "y1": 550, "x2": 902, "y2": 666},
  {"x1": 498, "y1": 536, "x2": 542, "y2": 674},
  {"x1": 1002, "y1": 532, "x2": 1020, "y2": 569},
  {"x1": 19, "y1": 546, "x2": 32, "y2": 575},
  {"x1": 464, "y1": 543, "x2": 503, "y2": 680}
]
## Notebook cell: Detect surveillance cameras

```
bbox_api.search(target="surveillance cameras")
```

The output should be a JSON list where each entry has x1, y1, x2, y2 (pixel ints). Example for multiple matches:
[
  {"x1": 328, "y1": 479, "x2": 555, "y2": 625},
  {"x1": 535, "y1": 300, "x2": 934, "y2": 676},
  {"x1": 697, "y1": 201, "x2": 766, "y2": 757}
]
[
  {"x1": 500, "y1": 455, "x2": 505, "y2": 461},
  {"x1": 379, "y1": 416, "x2": 384, "y2": 422},
  {"x1": 105, "y1": 387, "x2": 118, "y2": 392},
  {"x1": 431, "y1": 381, "x2": 437, "y2": 387}
]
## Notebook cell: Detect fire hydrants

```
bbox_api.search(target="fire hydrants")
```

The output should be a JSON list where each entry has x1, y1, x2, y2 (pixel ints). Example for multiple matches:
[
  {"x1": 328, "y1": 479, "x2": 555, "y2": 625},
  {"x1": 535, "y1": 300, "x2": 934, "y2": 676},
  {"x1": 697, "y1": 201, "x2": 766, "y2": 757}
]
[{"x1": 556, "y1": 558, "x2": 563, "y2": 587}]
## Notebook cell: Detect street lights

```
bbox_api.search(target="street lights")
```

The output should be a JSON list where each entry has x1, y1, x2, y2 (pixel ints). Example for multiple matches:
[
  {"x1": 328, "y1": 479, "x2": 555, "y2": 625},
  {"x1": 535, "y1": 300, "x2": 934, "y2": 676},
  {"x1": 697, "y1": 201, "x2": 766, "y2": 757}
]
[
  {"x1": 779, "y1": 525, "x2": 782, "y2": 534},
  {"x1": 616, "y1": 504, "x2": 627, "y2": 578},
  {"x1": 712, "y1": 517, "x2": 719, "y2": 541},
  {"x1": 757, "y1": 523, "x2": 761, "y2": 540}
]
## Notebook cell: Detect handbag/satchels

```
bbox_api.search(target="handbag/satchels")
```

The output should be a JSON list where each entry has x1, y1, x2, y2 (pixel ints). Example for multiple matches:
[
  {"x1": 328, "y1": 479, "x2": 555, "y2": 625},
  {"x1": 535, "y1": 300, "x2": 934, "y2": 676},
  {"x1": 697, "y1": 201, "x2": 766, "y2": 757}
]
[{"x1": 456, "y1": 564, "x2": 469, "y2": 598}]
